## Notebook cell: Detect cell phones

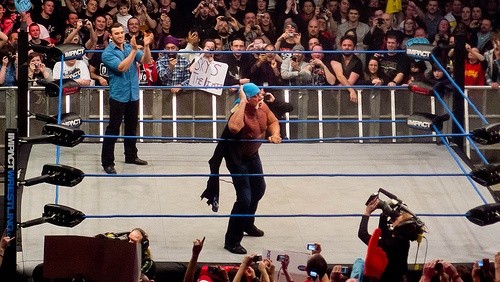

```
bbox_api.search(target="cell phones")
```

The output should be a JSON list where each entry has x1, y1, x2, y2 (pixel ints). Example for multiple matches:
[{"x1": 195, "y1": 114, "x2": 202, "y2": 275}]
[
  {"x1": 221, "y1": 17, "x2": 231, "y2": 21},
  {"x1": 477, "y1": 260, "x2": 485, "y2": 267},
  {"x1": 277, "y1": 255, "x2": 286, "y2": 261},
  {"x1": 307, "y1": 243, "x2": 316, "y2": 250},
  {"x1": 191, "y1": 29, "x2": 195, "y2": 36},
  {"x1": 169, "y1": 54, "x2": 174, "y2": 58}
]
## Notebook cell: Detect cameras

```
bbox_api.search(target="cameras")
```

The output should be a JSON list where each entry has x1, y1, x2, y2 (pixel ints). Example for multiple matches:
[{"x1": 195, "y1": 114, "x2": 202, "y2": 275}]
[
  {"x1": 203, "y1": 2, "x2": 208, "y2": 7},
  {"x1": 435, "y1": 260, "x2": 445, "y2": 272},
  {"x1": 340, "y1": 266, "x2": 349, "y2": 273},
  {"x1": 209, "y1": 49, "x2": 214, "y2": 51},
  {"x1": 287, "y1": 33, "x2": 294, "y2": 38},
  {"x1": 6, "y1": 53, "x2": 16, "y2": 63},
  {"x1": 319, "y1": 6, "x2": 326, "y2": 13},
  {"x1": 139, "y1": 1, "x2": 143, "y2": 6},
  {"x1": 82, "y1": 21, "x2": 87, "y2": 26},
  {"x1": 475, "y1": 21, "x2": 481, "y2": 25},
  {"x1": 439, "y1": 32, "x2": 444, "y2": 38},
  {"x1": 251, "y1": 25, "x2": 257, "y2": 30},
  {"x1": 264, "y1": 94, "x2": 270, "y2": 100},
  {"x1": 208, "y1": 266, "x2": 219, "y2": 274},
  {"x1": 376, "y1": 18, "x2": 384, "y2": 24},
  {"x1": 162, "y1": 8, "x2": 167, "y2": 14},
  {"x1": 254, "y1": 256, "x2": 262, "y2": 261},
  {"x1": 257, "y1": 13, "x2": 264, "y2": 19}
]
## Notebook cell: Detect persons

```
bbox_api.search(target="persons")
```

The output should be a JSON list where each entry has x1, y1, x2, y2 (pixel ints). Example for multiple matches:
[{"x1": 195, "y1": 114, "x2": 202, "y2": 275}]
[
  {"x1": 259, "y1": 88, "x2": 293, "y2": 142},
  {"x1": 358, "y1": 195, "x2": 424, "y2": 282},
  {"x1": 220, "y1": 82, "x2": 283, "y2": 254},
  {"x1": 0, "y1": 226, "x2": 500, "y2": 282},
  {"x1": 100, "y1": 24, "x2": 155, "y2": 175},
  {"x1": 1, "y1": 0, "x2": 499, "y2": 103}
]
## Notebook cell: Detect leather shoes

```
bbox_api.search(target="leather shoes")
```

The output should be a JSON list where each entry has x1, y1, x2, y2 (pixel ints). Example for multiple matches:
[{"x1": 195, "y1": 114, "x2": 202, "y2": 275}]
[
  {"x1": 102, "y1": 162, "x2": 117, "y2": 174},
  {"x1": 125, "y1": 157, "x2": 148, "y2": 165},
  {"x1": 224, "y1": 223, "x2": 264, "y2": 254}
]
